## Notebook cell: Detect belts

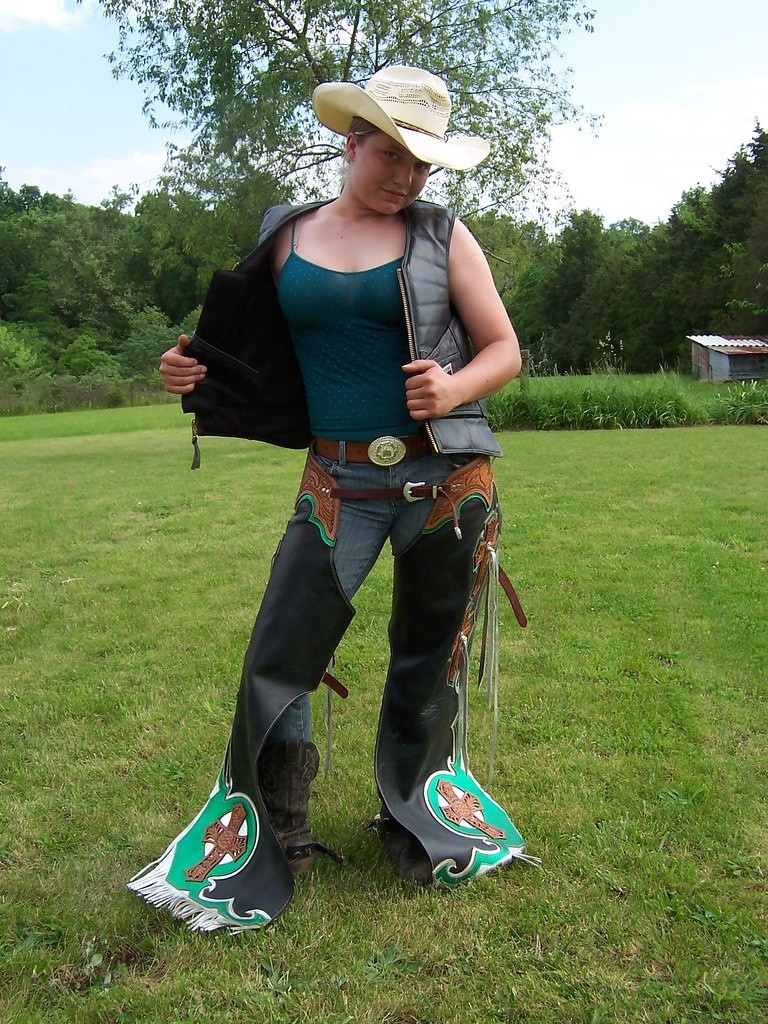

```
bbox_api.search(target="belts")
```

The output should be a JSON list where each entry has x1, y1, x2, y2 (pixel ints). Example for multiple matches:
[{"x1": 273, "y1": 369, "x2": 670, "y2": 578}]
[
  {"x1": 330, "y1": 482, "x2": 462, "y2": 541},
  {"x1": 312, "y1": 436, "x2": 433, "y2": 465}
]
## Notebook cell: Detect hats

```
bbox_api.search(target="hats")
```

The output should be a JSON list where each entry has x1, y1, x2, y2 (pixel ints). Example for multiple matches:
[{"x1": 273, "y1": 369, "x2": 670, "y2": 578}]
[{"x1": 312, "y1": 67, "x2": 490, "y2": 170}]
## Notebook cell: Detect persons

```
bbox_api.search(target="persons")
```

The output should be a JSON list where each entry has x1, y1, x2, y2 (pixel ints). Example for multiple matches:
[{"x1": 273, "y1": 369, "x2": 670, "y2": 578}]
[{"x1": 124, "y1": 65, "x2": 542, "y2": 936}]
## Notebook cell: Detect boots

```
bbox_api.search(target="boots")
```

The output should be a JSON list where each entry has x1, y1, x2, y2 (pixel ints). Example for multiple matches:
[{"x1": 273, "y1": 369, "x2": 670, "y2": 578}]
[
  {"x1": 376, "y1": 803, "x2": 434, "y2": 884},
  {"x1": 256, "y1": 740, "x2": 320, "y2": 873}
]
